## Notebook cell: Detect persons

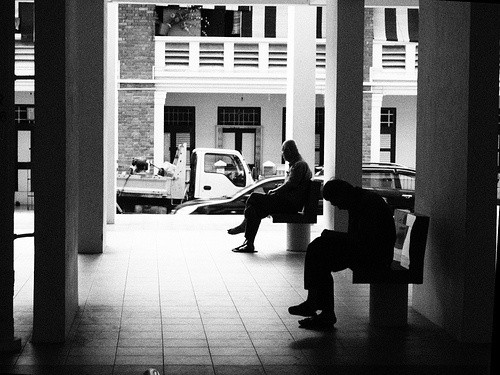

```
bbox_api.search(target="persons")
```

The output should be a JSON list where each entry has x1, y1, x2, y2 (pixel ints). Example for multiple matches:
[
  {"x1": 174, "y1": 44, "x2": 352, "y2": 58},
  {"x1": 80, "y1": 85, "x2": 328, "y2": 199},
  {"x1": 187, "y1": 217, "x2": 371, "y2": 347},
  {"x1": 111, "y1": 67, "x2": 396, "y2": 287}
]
[
  {"x1": 228, "y1": 139, "x2": 311, "y2": 253},
  {"x1": 289, "y1": 178, "x2": 397, "y2": 328}
]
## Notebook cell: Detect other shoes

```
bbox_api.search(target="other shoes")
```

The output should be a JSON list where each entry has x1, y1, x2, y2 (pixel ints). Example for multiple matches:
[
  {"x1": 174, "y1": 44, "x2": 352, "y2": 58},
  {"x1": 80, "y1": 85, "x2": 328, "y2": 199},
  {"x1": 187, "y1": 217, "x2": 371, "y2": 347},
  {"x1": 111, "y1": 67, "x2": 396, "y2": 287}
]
[
  {"x1": 227, "y1": 225, "x2": 245, "y2": 234},
  {"x1": 233, "y1": 244, "x2": 258, "y2": 252},
  {"x1": 298, "y1": 316, "x2": 335, "y2": 329},
  {"x1": 289, "y1": 302, "x2": 318, "y2": 317}
]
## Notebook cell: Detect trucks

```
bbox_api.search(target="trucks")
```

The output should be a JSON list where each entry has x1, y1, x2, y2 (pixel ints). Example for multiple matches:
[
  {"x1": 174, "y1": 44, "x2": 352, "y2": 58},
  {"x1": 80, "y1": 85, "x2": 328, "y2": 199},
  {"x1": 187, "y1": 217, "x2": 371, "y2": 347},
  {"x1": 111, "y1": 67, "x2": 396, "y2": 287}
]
[{"x1": 117, "y1": 142, "x2": 265, "y2": 200}]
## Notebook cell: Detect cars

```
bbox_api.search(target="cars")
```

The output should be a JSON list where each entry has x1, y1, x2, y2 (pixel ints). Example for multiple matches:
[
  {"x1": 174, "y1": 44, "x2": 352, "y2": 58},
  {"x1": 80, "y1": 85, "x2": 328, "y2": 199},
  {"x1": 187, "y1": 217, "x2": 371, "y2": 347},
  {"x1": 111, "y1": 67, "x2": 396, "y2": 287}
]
[{"x1": 170, "y1": 174, "x2": 325, "y2": 215}]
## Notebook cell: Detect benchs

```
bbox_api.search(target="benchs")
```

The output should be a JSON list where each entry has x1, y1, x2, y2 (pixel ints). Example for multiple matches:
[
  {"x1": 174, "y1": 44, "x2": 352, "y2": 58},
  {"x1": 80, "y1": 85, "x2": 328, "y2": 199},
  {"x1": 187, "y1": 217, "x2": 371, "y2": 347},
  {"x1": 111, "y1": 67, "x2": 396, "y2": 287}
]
[
  {"x1": 353, "y1": 209, "x2": 430, "y2": 327},
  {"x1": 273, "y1": 180, "x2": 322, "y2": 252}
]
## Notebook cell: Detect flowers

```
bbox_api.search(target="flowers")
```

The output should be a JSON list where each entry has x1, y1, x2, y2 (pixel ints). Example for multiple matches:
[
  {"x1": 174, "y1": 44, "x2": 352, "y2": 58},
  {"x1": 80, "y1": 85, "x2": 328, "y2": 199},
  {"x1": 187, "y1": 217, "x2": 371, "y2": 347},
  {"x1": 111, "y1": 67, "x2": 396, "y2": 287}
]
[{"x1": 167, "y1": 6, "x2": 210, "y2": 36}]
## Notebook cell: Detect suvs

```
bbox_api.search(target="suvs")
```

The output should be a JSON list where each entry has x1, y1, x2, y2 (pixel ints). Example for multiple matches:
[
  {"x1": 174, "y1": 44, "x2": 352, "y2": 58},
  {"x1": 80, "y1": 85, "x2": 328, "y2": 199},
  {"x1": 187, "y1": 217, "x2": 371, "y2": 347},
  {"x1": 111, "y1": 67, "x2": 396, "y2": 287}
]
[{"x1": 315, "y1": 161, "x2": 416, "y2": 216}]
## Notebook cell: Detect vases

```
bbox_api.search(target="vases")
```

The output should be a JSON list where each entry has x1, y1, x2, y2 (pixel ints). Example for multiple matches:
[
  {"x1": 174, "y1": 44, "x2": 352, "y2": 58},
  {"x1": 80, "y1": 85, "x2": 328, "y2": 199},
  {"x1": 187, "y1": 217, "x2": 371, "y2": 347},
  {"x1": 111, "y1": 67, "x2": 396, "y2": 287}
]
[{"x1": 159, "y1": 23, "x2": 170, "y2": 35}]
